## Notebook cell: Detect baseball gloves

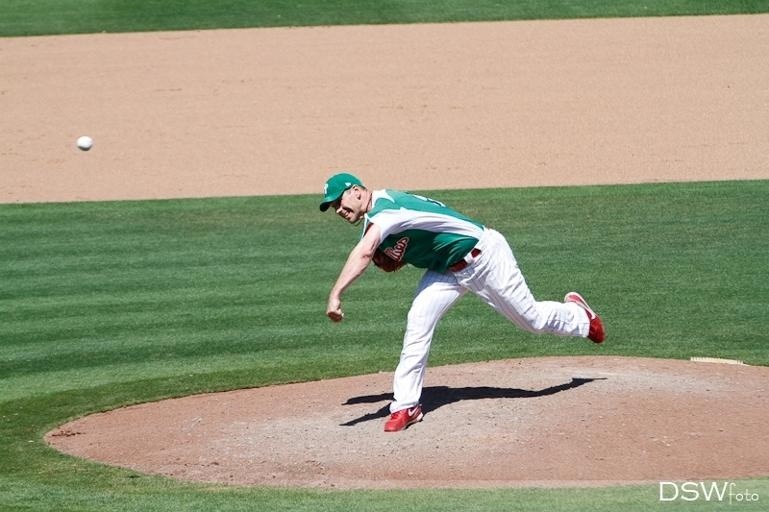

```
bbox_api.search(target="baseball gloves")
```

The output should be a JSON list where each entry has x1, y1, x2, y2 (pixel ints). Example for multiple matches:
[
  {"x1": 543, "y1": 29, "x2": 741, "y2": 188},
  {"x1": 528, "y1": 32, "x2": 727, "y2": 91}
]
[{"x1": 371, "y1": 248, "x2": 407, "y2": 273}]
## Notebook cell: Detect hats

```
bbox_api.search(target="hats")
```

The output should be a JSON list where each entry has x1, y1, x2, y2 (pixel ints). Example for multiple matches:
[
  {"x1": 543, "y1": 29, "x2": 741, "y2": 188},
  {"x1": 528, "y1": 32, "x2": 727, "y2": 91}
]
[{"x1": 319, "y1": 174, "x2": 364, "y2": 212}]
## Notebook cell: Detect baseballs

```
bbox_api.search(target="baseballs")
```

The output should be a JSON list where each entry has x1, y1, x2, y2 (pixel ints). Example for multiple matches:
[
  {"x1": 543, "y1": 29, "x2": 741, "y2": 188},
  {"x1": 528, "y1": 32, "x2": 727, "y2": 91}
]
[{"x1": 76, "y1": 135, "x2": 93, "y2": 151}]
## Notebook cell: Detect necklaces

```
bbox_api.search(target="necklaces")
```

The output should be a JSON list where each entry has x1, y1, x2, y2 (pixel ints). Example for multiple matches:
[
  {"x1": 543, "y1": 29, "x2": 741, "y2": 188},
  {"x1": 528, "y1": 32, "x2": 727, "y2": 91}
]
[{"x1": 353, "y1": 191, "x2": 374, "y2": 228}]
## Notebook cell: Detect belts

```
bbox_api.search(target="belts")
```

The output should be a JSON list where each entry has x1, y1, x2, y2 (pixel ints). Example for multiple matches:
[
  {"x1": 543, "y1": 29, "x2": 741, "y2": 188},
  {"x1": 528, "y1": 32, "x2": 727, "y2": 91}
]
[{"x1": 448, "y1": 249, "x2": 481, "y2": 272}]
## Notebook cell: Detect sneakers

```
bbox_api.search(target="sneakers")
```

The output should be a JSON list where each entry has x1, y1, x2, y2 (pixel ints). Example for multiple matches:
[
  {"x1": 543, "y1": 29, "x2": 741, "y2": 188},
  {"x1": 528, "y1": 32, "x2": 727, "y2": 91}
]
[
  {"x1": 383, "y1": 405, "x2": 424, "y2": 432},
  {"x1": 564, "y1": 291, "x2": 604, "y2": 342}
]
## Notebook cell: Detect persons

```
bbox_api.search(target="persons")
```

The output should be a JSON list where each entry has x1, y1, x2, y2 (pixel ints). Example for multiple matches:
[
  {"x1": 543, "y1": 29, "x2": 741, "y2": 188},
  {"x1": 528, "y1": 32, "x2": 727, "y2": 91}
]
[{"x1": 318, "y1": 172, "x2": 607, "y2": 433}]
